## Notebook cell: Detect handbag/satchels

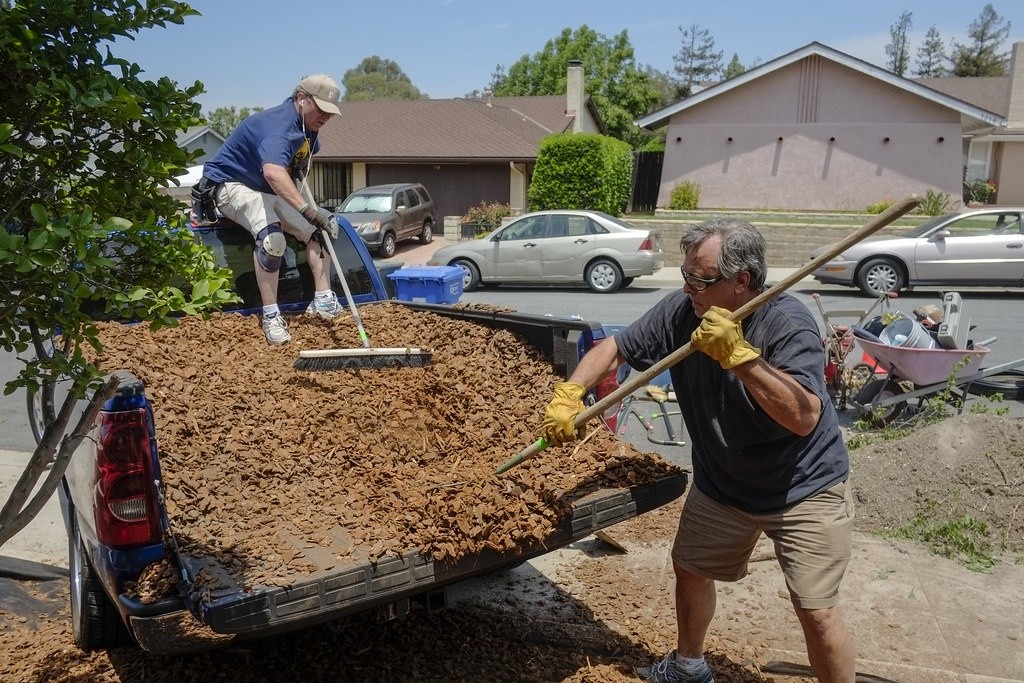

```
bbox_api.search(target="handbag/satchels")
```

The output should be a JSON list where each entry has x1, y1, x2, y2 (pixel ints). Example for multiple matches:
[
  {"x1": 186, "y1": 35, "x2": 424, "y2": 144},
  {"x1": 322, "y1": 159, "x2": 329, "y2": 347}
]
[{"x1": 190, "y1": 181, "x2": 217, "y2": 223}]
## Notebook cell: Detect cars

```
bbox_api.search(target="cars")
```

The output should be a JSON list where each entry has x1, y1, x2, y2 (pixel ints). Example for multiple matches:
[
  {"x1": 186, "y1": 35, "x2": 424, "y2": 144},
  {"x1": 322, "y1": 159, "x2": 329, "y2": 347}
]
[
  {"x1": 333, "y1": 183, "x2": 437, "y2": 257},
  {"x1": 801, "y1": 206, "x2": 1023, "y2": 299},
  {"x1": 427, "y1": 208, "x2": 665, "y2": 294}
]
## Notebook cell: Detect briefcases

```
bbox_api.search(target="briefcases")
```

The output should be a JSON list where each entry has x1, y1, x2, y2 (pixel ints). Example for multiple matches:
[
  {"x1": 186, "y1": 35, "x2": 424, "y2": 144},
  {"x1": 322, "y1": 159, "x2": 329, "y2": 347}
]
[{"x1": 938, "y1": 291, "x2": 971, "y2": 350}]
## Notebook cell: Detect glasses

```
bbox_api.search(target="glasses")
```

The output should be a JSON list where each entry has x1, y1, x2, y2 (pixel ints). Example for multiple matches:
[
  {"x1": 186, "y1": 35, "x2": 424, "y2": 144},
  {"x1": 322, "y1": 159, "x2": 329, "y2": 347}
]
[{"x1": 681, "y1": 265, "x2": 724, "y2": 291}]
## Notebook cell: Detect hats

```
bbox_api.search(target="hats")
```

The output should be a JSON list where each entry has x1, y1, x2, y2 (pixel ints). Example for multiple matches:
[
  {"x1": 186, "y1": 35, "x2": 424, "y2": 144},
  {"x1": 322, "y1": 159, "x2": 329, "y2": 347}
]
[{"x1": 298, "y1": 74, "x2": 343, "y2": 119}]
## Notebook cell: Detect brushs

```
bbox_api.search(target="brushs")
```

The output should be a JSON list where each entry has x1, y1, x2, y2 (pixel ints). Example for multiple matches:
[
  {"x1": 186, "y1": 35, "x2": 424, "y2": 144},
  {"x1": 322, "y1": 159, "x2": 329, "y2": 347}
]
[{"x1": 290, "y1": 166, "x2": 437, "y2": 371}]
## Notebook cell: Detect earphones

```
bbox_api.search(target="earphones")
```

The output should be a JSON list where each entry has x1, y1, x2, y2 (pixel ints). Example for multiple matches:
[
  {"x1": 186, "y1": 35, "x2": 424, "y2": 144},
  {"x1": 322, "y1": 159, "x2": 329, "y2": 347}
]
[{"x1": 301, "y1": 99, "x2": 304, "y2": 106}]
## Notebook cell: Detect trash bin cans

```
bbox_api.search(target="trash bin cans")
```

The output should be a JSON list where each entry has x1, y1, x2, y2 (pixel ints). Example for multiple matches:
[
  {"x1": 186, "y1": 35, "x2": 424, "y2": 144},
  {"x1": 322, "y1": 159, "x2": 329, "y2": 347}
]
[
  {"x1": 374, "y1": 260, "x2": 406, "y2": 300},
  {"x1": 386, "y1": 265, "x2": 468, "y2": 305}
]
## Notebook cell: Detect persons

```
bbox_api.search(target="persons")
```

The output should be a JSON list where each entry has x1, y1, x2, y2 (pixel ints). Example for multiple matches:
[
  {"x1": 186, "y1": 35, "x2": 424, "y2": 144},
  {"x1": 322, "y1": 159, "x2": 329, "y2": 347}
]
[
  {"x1": 200, "y1": 73, "x2": 343, "y2": 348},
  {"x1": 542, "y1": 218, "x2": 857, "y2": 683}
]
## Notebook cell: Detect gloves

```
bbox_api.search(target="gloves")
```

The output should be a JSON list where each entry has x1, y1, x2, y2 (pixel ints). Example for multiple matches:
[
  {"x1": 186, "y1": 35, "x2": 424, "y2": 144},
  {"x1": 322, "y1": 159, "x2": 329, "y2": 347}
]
[
  {"x1": 691, "y1": 306, "x2": 762, "y2": 371},
  {"x1": 542, "y1": 382, "x2": 587, "y2": 449},
  {"x1": 299, "y1": 204, "x2": 332, "y2": 238}
]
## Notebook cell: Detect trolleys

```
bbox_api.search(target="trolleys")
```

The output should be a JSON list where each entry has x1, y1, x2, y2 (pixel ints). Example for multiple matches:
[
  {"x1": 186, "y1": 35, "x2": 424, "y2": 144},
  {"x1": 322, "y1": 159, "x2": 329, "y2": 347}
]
[{"x1": 851, "y1": 323, "x2": 999, "y2": 427}]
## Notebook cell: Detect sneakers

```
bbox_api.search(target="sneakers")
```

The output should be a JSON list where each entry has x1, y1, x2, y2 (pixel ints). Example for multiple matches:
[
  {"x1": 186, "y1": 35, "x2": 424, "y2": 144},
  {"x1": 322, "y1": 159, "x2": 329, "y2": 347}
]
[
  {"x1": 305, "y1": 291, "x2": 345, "y2": 319},
  {"x1": 631, "y1": 649, "x2": 715, "y2": 683},
  {"x1": 261, "y1": 311, "x2": 291, "y2": 347}
]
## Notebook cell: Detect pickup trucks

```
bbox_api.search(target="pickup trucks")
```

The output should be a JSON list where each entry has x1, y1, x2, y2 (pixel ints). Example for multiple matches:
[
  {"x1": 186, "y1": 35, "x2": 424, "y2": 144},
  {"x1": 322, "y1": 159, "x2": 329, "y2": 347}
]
[{"x1": 26, "y1": 215, "x2": 690, "y2": 660}]
